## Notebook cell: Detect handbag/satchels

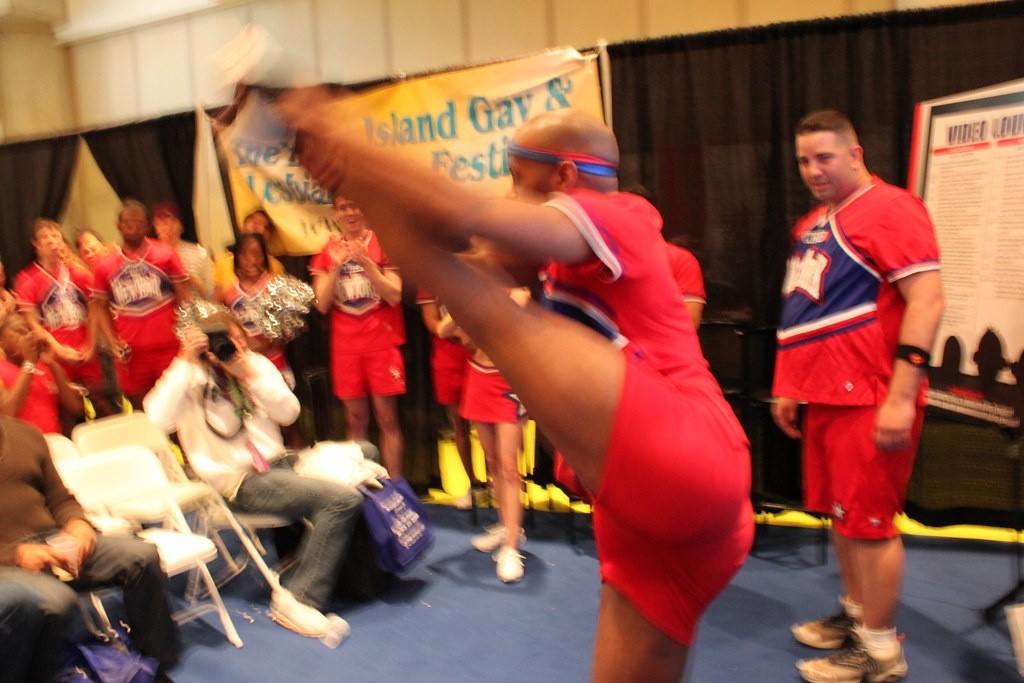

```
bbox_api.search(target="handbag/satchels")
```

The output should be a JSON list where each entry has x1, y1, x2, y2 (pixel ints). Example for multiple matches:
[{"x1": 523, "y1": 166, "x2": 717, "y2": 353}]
[
  {"x1": 360, "y1": 476, "x2": 435, "y2": 579},
  {"x1": 71, "y1": 626, "x2": 173, "y2": 683}
]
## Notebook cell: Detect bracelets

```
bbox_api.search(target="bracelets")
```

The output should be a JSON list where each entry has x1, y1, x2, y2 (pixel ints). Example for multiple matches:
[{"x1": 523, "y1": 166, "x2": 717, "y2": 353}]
[
  {"x1": 21, "y1": 362, "x2": 45, "y2": 376},
  {"x1": 896, "y1": 345, "x2": 930, "y2": 368}
]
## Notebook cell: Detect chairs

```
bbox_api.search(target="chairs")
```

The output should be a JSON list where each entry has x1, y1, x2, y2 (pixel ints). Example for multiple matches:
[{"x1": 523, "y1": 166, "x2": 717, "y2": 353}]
[{"x1": 44, "y1": 411, "x2": 312, "y2": 659}]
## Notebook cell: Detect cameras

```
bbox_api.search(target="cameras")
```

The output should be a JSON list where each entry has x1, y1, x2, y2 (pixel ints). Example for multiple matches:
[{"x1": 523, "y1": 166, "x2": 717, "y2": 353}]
[{"x1": 199, "y1": 321, "x2": 235, "y2": 361}]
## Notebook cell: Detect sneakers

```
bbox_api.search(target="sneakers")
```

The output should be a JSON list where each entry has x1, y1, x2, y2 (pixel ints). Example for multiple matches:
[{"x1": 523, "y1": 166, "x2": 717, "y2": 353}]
[
  {"x1": 270, "y1": 583, "x2": 335, "y2": 638},
  {"x1": 796, "y1": 633, "x2": 908, "y2": 681},
  {"x1": 470, "y1": 525, "x2": 528, "y2": 552},
  {"x1": 792, "y1": 593, "x2": 906, "y2": 646},
  {"x1": 493, "y1": 546, "x2": 526, "y2": 582}
]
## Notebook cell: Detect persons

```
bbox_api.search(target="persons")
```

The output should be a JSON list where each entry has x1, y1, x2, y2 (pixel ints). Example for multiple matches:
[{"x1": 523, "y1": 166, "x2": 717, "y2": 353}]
[
  {"x1": 1, "y1": 199, "x2": 704, "y2": 504},
  {"x1": 772, "y1": 109, "x2": 946, "y2": 683},
  {"x1": 15, "y1": 218, "x2": 110, "y2": 440},
  {"x1": 1, "y1": 414, "x2": 180, "y2": 682},
  {"x1": 199, "y1": 26, "x2": 755, "y2": 683},
  {"x1": 309, "y1": 191, "x2": 406, "y2": 481},
  {"x1": 436, "y1": 248, "x2": 532, "y2": 583},
  {"x1": 91, "y1": 201, "x2": 191, "y2": 413},
  {"x1": 143, "y1": 302, "x2": 391, "y2": 639}
]
